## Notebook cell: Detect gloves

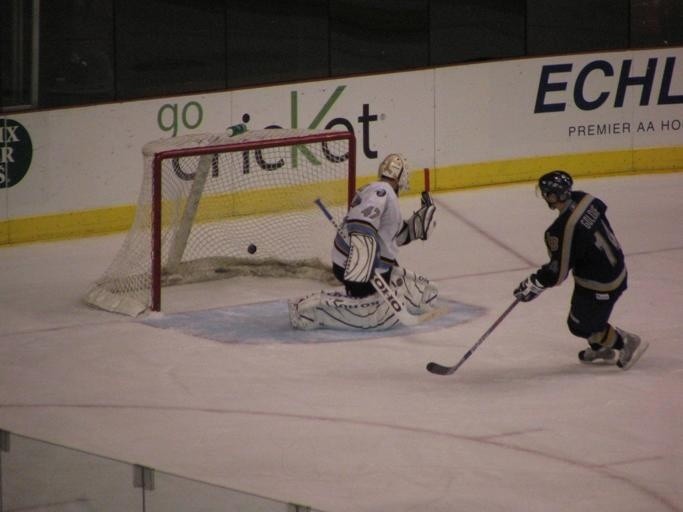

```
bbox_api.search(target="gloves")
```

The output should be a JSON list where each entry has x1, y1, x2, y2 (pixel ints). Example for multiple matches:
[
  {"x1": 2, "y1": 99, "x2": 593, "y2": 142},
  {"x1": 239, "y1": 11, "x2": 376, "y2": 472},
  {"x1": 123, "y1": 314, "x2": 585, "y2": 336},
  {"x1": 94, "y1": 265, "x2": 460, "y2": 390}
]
[{"x1": 511, "y1": 273, "x2": 549, "y2": 303}]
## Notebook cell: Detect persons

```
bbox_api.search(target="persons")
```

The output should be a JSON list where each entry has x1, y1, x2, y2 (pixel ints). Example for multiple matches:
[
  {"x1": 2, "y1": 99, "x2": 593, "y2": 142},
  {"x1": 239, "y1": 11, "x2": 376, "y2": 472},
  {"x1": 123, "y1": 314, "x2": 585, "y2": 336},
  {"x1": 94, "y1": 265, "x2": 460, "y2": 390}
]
[
  {"x1": 509, "y1": 169, "x2": 648, "y2": 371},
  {"x1": 284, "y1": 153, "x2": 439, "y2": 332}
]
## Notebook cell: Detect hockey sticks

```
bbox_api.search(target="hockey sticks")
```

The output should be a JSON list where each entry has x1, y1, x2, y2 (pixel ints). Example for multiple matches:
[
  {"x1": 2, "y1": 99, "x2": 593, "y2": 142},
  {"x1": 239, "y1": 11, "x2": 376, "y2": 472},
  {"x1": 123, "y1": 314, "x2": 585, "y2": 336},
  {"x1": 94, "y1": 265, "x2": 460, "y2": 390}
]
[
  {"x1": 315, "y1": 197, "x2": 449, "y2": 328},
  {"x1": 427, "y1": 298, "x2": 522, "y2": 375}
]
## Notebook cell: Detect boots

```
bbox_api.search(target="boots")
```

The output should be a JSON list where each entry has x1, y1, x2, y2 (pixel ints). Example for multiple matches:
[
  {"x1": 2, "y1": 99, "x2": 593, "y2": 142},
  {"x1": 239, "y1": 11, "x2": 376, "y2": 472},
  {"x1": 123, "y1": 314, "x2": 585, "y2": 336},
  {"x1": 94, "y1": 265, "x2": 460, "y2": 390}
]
[
  {"x1": 613, "y1": 325, "x2": 641, "y2": 368},
  {"x1": 576, "y1": 345, "x2": 615, "y2": 361}
]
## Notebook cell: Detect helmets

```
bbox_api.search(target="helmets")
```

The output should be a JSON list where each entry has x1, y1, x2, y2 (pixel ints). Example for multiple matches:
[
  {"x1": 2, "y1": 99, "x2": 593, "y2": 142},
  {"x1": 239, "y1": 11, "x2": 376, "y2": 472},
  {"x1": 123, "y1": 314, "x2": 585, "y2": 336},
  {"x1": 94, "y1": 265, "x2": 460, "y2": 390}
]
[
  {"x1": 535, "y1": 168, "x2": 575, "y2": 202},
  {"x1": 377, "y1": 152, "x2": 410, "y2": 183}
]
